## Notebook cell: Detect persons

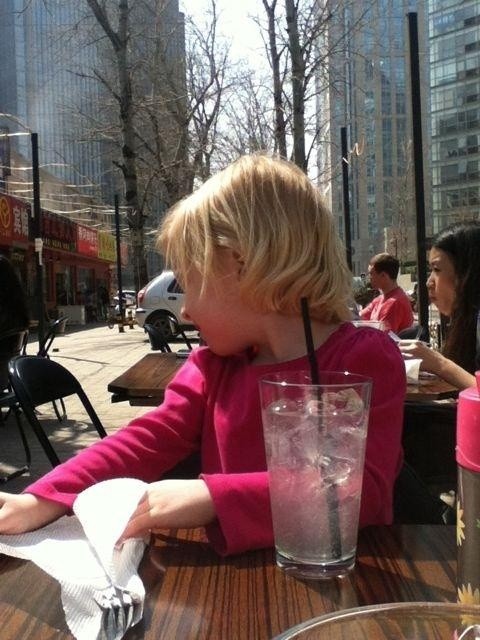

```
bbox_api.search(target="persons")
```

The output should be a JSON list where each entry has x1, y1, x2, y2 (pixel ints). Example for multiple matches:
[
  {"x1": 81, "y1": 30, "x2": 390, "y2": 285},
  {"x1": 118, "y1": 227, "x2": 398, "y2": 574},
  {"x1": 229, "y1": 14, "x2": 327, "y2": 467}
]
[
  {"x1": 0, "y1": 146, "x2": 407, "y2": 556},
  {"x1": 358, "y1": 253, "x2": 413, "y2": 334},
  {"x1": 101, "y1": 288, "x2": 109, "y2": 317},
  {"x1": 398, "y1": 224, "x2": 480, "y2": 393},
  {"x1": 2, "y1": 254, "x2": 30, "y2": 392}
]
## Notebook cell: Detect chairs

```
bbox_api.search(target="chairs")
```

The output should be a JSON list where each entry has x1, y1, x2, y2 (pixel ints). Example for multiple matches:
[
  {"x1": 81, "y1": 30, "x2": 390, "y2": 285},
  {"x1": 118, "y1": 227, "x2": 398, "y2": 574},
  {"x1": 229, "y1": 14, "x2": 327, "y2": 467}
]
[
  {"x1": 3, "y1": 315, "x2": 107, "y2": 487},
  {"x1": 143, "y1": 314, "x2": 193, "y2": 354}
]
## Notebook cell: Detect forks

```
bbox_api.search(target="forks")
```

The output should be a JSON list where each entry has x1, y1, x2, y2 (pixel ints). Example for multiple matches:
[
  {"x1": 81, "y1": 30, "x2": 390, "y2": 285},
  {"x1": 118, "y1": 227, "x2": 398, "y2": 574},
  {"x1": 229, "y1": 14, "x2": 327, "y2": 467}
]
[{"x1": 97, "y1": 587, "x2": 144, "y2": 640}]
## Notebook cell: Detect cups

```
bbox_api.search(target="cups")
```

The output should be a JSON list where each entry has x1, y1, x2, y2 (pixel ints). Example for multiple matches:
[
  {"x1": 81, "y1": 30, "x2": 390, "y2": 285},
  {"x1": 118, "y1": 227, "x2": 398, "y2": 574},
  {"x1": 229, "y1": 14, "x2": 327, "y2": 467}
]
[{"x1": 258, "y1": 369, "x2": 374, "y2": 580}]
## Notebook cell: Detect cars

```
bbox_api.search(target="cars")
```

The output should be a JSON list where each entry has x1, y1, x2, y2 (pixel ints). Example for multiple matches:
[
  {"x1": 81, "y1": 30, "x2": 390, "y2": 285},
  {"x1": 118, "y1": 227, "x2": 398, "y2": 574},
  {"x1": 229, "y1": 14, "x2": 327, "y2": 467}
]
[{"x1": 134, "y1": 270, "x2": 199, "y2": 342}]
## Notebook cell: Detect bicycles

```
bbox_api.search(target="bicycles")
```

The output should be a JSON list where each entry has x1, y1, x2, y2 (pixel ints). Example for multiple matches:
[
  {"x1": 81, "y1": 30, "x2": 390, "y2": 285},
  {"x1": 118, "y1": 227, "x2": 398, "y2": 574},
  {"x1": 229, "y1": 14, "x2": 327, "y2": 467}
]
[{"x1": 107, "y1": 305, "x2": 117, "y2": 329}]
